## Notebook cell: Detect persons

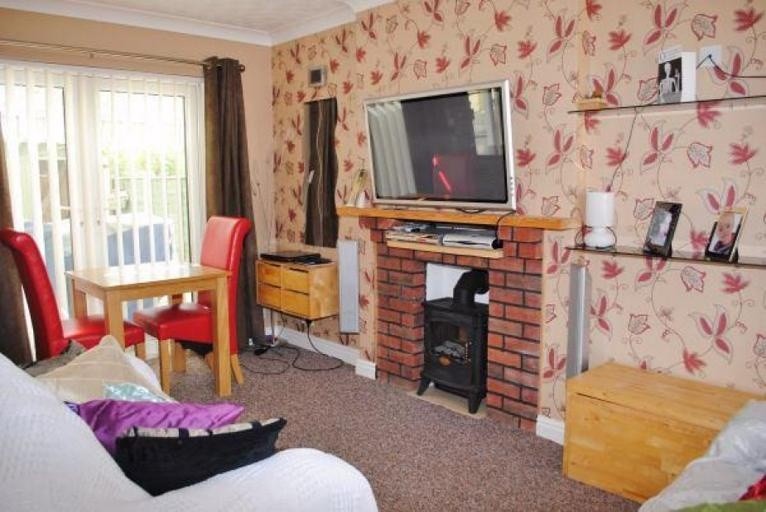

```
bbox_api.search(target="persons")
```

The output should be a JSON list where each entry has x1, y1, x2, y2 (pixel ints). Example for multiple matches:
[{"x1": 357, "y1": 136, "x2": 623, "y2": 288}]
[
  {"x1": 648, "y1": 211, "x2": 672, "y2": 246},
  {"x1": 712, "y1": 213, "x2": 735, "y2": 254}
]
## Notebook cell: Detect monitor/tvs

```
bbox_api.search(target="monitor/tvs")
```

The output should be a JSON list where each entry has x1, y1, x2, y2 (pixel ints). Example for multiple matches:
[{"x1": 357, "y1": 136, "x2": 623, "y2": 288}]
[{"x1": 361, "y1": 78, "x2": 518, "y2": 214}]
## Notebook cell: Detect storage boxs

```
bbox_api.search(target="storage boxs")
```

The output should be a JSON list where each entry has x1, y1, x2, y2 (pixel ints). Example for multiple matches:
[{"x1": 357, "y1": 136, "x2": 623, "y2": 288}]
[{"x1": 562, "y1": 359, "x2": 766, "y2": 503}]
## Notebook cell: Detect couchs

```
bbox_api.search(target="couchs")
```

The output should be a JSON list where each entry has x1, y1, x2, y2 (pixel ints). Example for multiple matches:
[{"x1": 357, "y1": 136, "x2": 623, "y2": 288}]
[{"x1": 1, "y1": 351, "x2": 378, "y2": 511}]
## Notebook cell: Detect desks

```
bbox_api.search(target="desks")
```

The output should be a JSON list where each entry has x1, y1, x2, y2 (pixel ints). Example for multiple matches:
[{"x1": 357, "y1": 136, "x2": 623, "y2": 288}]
[{"x1": 65, "y1": 259, "x2": 234, "y2": 397}]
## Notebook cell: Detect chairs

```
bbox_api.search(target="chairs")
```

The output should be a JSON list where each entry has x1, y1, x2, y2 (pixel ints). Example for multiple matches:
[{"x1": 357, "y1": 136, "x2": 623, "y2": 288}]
[
  {"x1": 1, "y1": 226, "x2": 147, "y2": 364},
  {"x1": 134, "y1": 214, "x2": 254, "y2": 383}
]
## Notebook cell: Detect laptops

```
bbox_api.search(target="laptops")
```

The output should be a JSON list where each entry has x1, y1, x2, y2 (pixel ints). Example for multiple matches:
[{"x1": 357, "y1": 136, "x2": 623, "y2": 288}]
[{"x1": 258, "y1": 249, "x2": 322, "y2": 263}]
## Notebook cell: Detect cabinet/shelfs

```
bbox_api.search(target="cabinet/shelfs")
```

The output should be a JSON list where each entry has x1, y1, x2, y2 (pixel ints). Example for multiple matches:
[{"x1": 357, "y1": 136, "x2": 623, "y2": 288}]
[
  {"x1": 566, "y1": 94, "x2": 765, "y2": 269},
  {"x1": 255, "y1": 260, "x2": 339, "y2": 321}
]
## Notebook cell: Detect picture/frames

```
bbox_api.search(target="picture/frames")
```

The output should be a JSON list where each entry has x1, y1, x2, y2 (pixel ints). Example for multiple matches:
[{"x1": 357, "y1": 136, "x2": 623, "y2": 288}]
[
  {"x1": 641, "y1": 201, "x2": 682, "y2": 260},
  {"x1": 702, "y1": 206, "x2": 749, "y2": 262}
]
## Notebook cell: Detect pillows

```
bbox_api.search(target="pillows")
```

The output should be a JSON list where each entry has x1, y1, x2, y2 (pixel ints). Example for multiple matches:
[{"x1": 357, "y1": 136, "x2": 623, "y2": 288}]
[{"x1": 37, "y1": 334, "x2": 288, "y2": 500}]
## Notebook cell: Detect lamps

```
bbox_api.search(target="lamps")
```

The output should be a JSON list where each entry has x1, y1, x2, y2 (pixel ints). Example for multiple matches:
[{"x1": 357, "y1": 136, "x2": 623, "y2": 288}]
[{"x1": 582, "y1": 191, "x2": 616, "y2": 249}]
